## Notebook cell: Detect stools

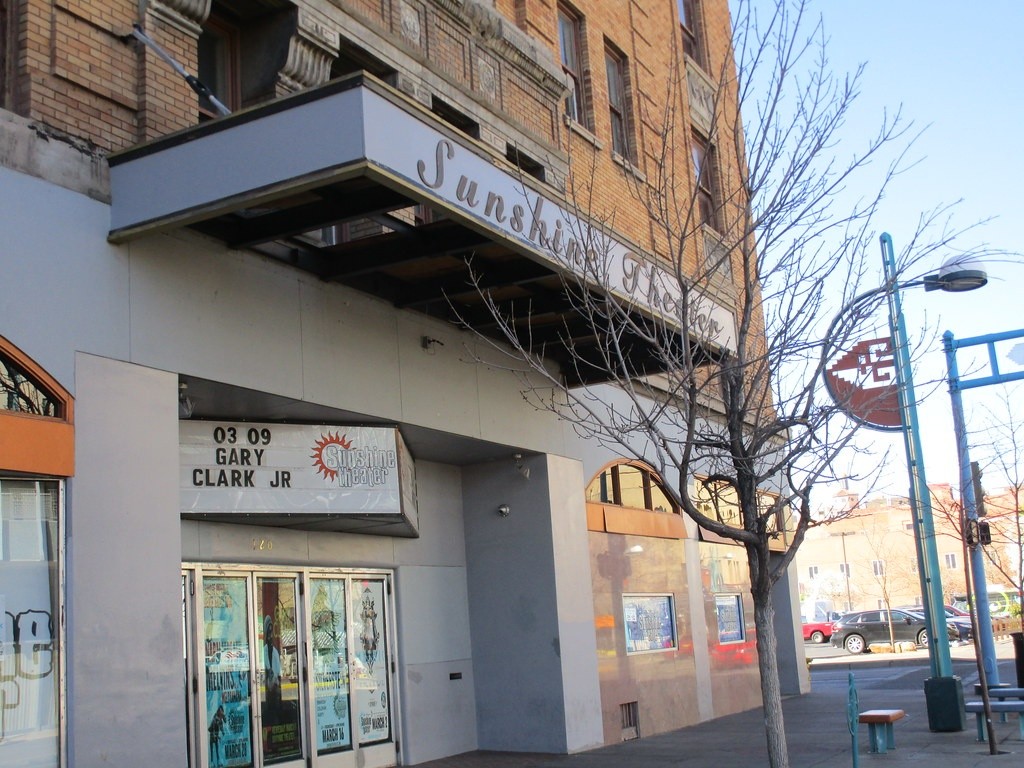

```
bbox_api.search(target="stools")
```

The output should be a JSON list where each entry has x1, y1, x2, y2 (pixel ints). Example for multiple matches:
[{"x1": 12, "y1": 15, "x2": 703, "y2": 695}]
[{"x1": 859, "y1": 709, "x2": 905, "y2": 754}]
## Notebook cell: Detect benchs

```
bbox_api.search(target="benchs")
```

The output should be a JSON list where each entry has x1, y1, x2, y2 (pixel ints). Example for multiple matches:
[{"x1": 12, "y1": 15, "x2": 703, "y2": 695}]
[
  {"x1": 988, "y1": 687, "x2": 1024, "y2": 724},
  {"x1": 965, "y1": 700, "x2": 1024, "y2": 742}
]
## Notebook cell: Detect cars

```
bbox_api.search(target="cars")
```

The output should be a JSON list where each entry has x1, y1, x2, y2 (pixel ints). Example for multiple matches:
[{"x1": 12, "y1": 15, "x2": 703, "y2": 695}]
[
  {"x1": 830, "y1": 610, "x2": 959, "y2": 655},
  {"x1": 802, "y1": 589, "x2": 1022, "y2": 644}
]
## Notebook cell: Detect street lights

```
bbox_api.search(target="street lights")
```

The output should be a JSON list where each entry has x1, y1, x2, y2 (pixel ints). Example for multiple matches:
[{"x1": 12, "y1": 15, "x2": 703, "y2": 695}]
[{"x1": 823, "y1": 231, "x2": 990, "y2": 734}]
